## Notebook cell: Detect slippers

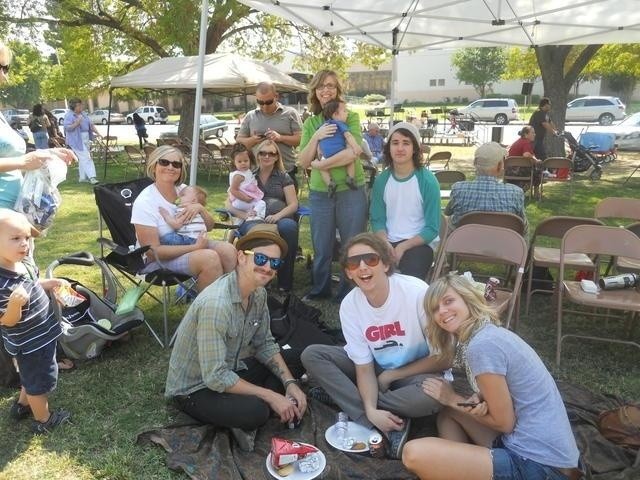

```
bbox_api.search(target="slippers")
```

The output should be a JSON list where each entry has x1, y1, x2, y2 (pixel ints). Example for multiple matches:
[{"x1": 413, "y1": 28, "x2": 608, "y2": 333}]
[{"x1": 57, "y1": 359, "x2": 75, "y2": 372}]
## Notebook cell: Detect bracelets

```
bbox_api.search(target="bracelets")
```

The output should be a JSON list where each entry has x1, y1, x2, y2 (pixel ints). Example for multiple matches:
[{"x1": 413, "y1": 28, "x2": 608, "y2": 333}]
[{"x1": 283, "y1": 378, "x2": 296, "y2": 391}]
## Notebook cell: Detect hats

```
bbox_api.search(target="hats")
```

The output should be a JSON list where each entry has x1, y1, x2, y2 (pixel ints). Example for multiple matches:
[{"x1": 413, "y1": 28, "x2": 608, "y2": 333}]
[
  {"x1": 474, "y1": 142, "x2": 507, "y2": 164},
  {"x1": 236, "y1": 224, "x2": 289, "y2": 260},
  {"x1": 387, "y1": 122, "x2": 421, "y2": 147}
]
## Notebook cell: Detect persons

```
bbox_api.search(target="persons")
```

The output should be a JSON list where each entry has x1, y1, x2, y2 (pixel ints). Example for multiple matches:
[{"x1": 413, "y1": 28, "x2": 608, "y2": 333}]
[
  {"x1": 164, "y1": 224, "x2": 308, "y2": 452},
  {"x1": 27, "y1": 104, "x2": 51, "y2": 149},
  {"x1": 401, "y1": 274, "x2": 584, "y2": 480},
  {"x1": 133, "y1": 113, "x2": 148, "y2": 149},
  {"x1": 130, "y1": 145, "x2": 237, "y2": 292},
  {"x1": 226, "y1": 70, "x2": 559, "y2": 304},
  {"x1": 44, "y1": 109, "x2": 63, "y2": 148},
  {"x1": 159, "y1": 185, "x2": 207, "y2": 257},
  {"x1": 0, "y1": 41, "x2": 78, "y2": 208},
  {"x1": 300, "y1": 232, "x2": 456, "y2": 460},
  {"x1": 0, "y1": 209, "x2": 71, "y2": 434},
  {"x1": 63, "y1": 98, "x2": 100, "y2": 185}
]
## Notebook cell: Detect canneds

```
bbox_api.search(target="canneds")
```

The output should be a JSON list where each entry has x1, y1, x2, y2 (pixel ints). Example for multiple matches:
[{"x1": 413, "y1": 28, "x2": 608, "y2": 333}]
[
  {"x1": 484, "y1": 276, "x2": 499, "y2": 302},
  {"x1": 369, "y1": 432, "x2": 386, "y2": 458}
]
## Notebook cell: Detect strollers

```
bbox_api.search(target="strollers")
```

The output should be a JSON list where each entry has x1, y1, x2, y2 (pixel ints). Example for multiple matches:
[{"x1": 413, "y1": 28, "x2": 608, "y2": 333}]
[{"x1": 555, "y1": 124, "x2": 607, "y2": 182}]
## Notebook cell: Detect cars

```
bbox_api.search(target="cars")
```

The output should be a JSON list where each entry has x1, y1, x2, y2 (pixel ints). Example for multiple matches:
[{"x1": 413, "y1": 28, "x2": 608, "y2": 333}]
[
  {"x1": 49, "y1": 108, "x2": 69, "y2": 125},
  {"x1": 566, "y1": 96, "x2": 626, "y2": 126},
  {"x1": 200, "y1": 114, "x2": 228, "y2": 137},
  {"x1": 88, "y1": 110, "x2": 125, "y2": 124},
  {"x1": 614, "y1": 112, "x2": 640, "y2": 152}
]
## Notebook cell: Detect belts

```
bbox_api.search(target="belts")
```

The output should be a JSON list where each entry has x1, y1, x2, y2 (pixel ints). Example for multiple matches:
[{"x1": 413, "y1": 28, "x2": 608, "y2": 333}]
[{"x1": 560, "y1": 466, "x2": 585, "y2": 480}]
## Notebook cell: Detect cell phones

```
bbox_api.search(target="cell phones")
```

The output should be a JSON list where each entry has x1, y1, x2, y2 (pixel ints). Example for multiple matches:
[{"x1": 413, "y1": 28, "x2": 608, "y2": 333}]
[
  {"x1": 456, "y1": 402, "x2": 477, "y2": 409},
  {"x1": 258, "y1": 134, "x2": 266, "y2": 139}
]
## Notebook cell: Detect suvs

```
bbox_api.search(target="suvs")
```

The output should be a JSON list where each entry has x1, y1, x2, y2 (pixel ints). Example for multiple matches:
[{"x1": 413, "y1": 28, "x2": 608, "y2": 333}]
[
  {"x1": 125, "y1": 106, "x2": 168, "y2": 124},
  {"x1": 3, "y1": 109, "x2": 29, "y2": 127},
  {"x1": 451, "y1": 98, "x2": 519, "y2": 125}
]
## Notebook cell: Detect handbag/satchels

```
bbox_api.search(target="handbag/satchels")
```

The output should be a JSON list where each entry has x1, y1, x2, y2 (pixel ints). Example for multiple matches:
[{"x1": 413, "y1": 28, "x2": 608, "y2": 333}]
[
  {"x1": 29, "y1": 119, "x2": 42, "y2": 133},
  {"x1": 598, "y1": 405, "x2": 640, "y2": 450}
]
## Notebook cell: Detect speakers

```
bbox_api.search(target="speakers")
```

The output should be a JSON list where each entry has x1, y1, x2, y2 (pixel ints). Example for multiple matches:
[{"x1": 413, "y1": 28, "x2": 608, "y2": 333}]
[{"x1": 491, "y1": 126, "x2": 504, "y2": 143}]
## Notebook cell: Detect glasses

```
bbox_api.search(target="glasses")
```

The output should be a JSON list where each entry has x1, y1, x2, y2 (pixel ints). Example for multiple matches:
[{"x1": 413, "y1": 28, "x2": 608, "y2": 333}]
[
  {"x1": 156, "y1": 159, "x2": 182, "y2": 168},
  {"x1": 259, "y1": 151, "x2": 277, "y2": 156},
  {"x1": 343, "y1": 252, "x2": 381, "y2": 270},
  {"x1": 317, "y1": 83, "x2": 337, "y2": 90},
  {"x1": 243, "y1": 249, "x2": 282, "y2": 269},
  {"x1": 257, "y1": 95, "x2": 278, "y2": 106}
]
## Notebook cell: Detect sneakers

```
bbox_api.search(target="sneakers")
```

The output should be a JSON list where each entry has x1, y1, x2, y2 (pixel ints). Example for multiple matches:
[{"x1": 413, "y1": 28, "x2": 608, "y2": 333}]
[
  {"x1": 345, "y1": 174, "x2": 358, "y2": 191},
  {"x1": 31, "y1": 412, "x2": 72, "y2": 436},
  {"x1": 308, "y1": 386, "x2": 340, "y2": 412},
  {"x1": 328, "y1": 179, "x2": 337, "y2": 200},
  {"x1": 542, "y1": 171, "x2": 557, "y2": 178},
  {"x1": 388, "y1": 417, "x2": 412, "y2": 459},
  {"x1": 90, "y1": 177, "x2": 100, "y2": 185},
  {"x1": 11, "y1": 399, "x2": 32, "y2": 421},
  {"x1": 232, "y1": 427, "x2": 258, "y2": 452}
]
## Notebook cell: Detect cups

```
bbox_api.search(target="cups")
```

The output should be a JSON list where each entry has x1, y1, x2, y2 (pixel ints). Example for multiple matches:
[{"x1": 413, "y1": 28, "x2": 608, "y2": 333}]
[
  {"x1": 599, "y1": 272, "x2": 640, "y2": 290},
  {"x1": 335, "y1": 412, "x2": 348, "y2": 432}
]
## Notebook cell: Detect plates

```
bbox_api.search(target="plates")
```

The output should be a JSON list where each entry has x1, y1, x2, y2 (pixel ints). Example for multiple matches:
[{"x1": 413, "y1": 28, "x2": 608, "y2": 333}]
[
  {"x1": 266, "y1": 442, "x2": 327, "y2": 480},
  {"x1": 325, "y1": 421, "x2": 382, "y2": 453}
]
